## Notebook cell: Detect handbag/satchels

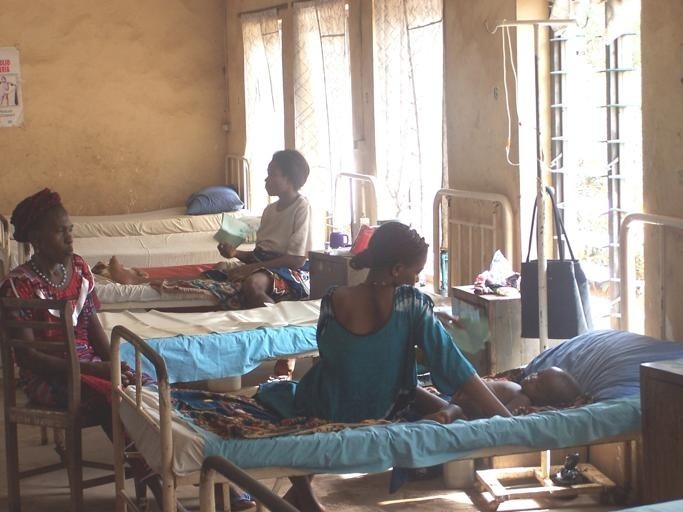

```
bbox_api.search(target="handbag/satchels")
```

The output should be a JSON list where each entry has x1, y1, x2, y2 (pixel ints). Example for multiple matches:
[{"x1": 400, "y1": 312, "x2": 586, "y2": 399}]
[{"x1": 519, "y1": 260, "x2": 593, "y2": 338}]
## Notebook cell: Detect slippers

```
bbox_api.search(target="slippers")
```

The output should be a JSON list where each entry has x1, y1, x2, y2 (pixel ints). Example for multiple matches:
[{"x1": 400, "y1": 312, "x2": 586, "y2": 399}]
[{"x1": 215, "y1": 492, "x2": 256, "y2": 511}]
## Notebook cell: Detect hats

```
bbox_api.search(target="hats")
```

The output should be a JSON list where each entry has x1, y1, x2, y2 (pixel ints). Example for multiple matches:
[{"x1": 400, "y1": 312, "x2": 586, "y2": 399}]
[{"x1": 10, "y1": 188, "x2": 60, "y2": 242}]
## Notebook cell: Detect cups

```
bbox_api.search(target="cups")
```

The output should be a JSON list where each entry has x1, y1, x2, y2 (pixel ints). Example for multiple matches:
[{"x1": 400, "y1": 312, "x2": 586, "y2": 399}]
[{"x1": 329, "y1": 231, "x2": 349, "y2": 249}]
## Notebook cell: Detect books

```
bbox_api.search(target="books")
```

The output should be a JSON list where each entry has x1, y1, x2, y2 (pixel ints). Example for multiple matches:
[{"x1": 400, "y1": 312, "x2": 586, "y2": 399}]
[{"x1": 213, "y1": 213, "x2": 250, "y2": 250}]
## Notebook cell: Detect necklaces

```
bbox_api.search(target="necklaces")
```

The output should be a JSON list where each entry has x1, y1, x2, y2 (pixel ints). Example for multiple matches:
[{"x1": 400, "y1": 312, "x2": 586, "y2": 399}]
[
  {"x1": 368, "y1": 280, "x2": 390, "y2": 286},
  {"x1": 30, "y1": 260, "x2": 67, "y2": 289}
]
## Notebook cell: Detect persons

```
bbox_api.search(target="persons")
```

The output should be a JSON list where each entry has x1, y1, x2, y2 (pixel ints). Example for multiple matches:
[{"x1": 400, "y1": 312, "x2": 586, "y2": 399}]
[
  {"x1": 413, "y1": 366, "x2": 583, "y2": 423},
  {"x1": 0, "y1": 76, "x2": 11, "y2": 106},
  {"x1": 0, "y1": 186, "x2": 189, "y2": 512},
  {"x1": 253, "y1": 219, "x2": 528, "y2": 512},
  {"x1": 105, "y1": 148, "x2": 312, "y2": 375}
]
